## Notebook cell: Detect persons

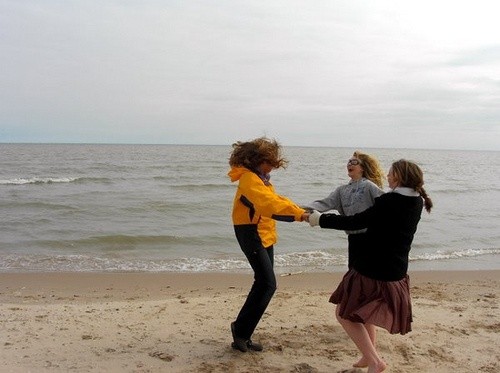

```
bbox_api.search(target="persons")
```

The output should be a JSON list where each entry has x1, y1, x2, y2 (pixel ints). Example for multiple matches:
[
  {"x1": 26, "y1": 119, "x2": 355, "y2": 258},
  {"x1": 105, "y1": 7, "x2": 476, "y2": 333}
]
[
  {"x1": 302, "y1": 151, "x2": 385, "y2": 270},
  {"x1": 308, "y1": 160, "x2": 433, "y2": 372},
  {"x1": 228, "y1": 137, "x2": 312, "y2": 353}
]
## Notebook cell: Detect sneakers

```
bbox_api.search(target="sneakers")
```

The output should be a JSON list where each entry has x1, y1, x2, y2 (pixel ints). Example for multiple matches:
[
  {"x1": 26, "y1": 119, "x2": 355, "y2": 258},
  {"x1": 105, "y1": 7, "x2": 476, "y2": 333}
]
[
  {"x1": 231, "y1": 322, "x2": 248, "y2": 352},
  {"x1": 231, "y1": 339, "x2": 263, "y2": 351}
]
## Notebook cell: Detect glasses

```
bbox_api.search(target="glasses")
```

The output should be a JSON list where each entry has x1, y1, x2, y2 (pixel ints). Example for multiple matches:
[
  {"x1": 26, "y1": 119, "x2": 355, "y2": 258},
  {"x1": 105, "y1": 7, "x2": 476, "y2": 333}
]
[{"x1": 347, "y1": 159, "x2": 364, "y2": 168}]
